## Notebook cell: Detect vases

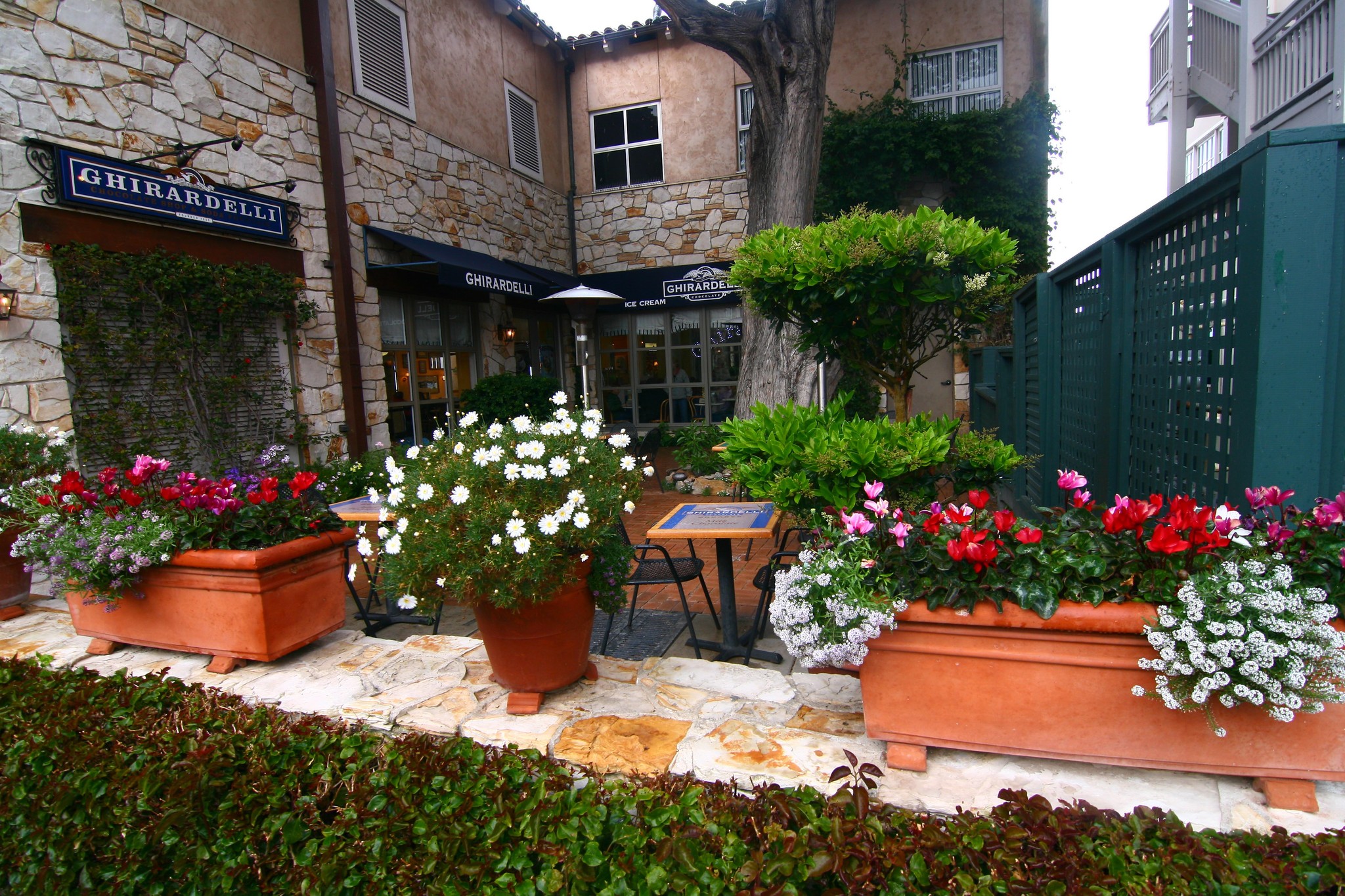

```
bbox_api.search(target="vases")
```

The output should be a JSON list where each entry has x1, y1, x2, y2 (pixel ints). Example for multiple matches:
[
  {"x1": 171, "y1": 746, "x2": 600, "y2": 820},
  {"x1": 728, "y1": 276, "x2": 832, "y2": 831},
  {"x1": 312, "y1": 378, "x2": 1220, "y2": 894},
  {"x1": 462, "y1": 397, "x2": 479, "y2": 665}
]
[
  {"x1": 464, "y1": 542, "x2": 601, "y2": 715},
  {"x1": 0, "y1": 503, "x2": 37, "y2": 613},
  {"x1": 853, "y1": 591, "x2": 1345, "y2": 814},
  {"x1": 63, "y1": 528, "x2": 354, "y2": 676}
]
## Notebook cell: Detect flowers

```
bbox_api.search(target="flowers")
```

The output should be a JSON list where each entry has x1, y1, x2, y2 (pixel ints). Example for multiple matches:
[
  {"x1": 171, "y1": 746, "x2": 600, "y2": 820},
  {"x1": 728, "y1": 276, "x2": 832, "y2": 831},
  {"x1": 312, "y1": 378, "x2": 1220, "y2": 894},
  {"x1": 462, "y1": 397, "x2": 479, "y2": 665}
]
[
  {"x1": 763, "y1": 467, "x2": 1344, "y2": 743},
  {"x1": 0, "y1": 453, "x2": 345, "y2": 613},
  {"x1": 0, "y1": 422, "x2": 75, "y2": 505},
  {"x1": 341, "y1": 389, "x2": 647, "y2": 620}
]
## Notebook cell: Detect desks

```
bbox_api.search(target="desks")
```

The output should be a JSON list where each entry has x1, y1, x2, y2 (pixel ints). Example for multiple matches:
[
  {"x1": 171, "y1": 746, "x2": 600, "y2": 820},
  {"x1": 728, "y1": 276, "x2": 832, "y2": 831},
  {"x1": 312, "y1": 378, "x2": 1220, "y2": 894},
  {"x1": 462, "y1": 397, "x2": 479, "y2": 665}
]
[
  {"x1": 646, "y1": 502, "x2": 784, "y2": 664},
  {"x1": 594, "y1": 432, "x2": 625, "y2": 440},
  {"x1": 326, "y1": 493, "x2": 445, "y2": 640},
  {"x1": 695, "y1": 403, "x2": 724, "y2": 415},
  {"x1": 622, "y1": 406, "x2": 641, "y2": 422}
]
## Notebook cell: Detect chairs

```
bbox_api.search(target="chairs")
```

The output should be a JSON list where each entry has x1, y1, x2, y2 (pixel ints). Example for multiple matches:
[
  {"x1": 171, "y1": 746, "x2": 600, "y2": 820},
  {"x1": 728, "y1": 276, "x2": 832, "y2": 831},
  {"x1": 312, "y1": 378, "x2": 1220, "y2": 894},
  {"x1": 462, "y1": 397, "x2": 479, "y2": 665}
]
[
  {"x1": 732, "y1": 475, "x2": 818, "y2": 667},
  {"x1": 649, "y1": 394, "x2": 706, "y2": 422},
  {"x1": 599, "y1": 510, "x2": 721, "y2": 659},
  {"x1": 606, "y1": 421, "x2": 665, "y2": 493},
  {"x1": 602, "y1": 398, "x2": 628, "y2": 424}
]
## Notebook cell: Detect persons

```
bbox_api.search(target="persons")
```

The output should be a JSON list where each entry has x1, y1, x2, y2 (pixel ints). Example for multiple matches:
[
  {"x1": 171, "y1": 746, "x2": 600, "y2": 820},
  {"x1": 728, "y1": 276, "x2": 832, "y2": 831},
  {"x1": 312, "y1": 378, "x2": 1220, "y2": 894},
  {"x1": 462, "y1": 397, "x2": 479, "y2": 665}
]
[{"x1": 605, "y1": 356, "x2": 694, "y2": 423}]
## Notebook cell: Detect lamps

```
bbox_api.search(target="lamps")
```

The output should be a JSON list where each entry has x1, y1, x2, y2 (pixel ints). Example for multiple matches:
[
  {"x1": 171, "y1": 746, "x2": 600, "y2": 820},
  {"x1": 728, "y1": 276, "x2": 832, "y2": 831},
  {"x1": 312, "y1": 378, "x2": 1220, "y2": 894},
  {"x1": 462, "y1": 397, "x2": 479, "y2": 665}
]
[
  {"x1": 517, "y1": 2, "x2": 669, "y2": 50},
  {"x1": 0, "y1": 274, "x2": 17, "y2": 321},
  {"x1": 241, "y1": 179, "x2": 297, "y2": 193},
  {"x1": 126, "y1": 134, "x2": 243, "y2": 169},
  {"x1": 497, "y1": 311, "x2": 517, "y2": 343}
]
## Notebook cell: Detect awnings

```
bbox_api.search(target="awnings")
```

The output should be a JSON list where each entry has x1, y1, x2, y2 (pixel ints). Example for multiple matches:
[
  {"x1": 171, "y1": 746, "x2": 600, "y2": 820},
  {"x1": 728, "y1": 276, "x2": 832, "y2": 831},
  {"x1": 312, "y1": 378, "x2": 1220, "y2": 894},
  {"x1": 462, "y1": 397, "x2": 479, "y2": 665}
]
[
  {"x1": 363, "y1": 223, "x2": 550, "y2": 288},
  {"x1": 503, "y1": 257, "x2": 579, "y2": 289}
]
